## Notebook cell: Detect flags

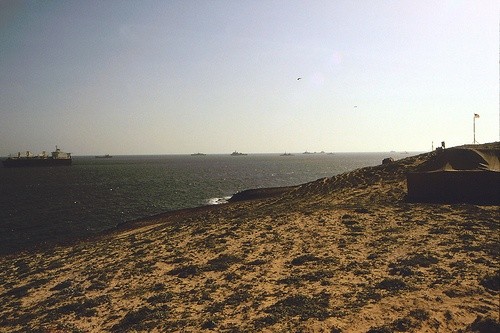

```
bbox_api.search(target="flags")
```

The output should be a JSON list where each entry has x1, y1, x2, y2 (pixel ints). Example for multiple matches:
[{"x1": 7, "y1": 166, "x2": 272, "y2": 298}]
[{"x1": 475, "y1": 114, "x2": 480, "y2": 118}]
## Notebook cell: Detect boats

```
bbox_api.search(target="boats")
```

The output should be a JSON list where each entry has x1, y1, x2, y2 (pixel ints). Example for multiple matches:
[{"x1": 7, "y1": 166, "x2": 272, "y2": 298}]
[
  {"x1": 281, "y1": 152, "x2": 294, "y2": 156},
  {"x1": 230, "y1": 150, "x2": 247, "y2": 155},
  {"x1": 190, "y1": 153, "x2": 207, "y2": 156},
  {"x1": 302, "y1": 151, "x2": 333, "y2": 155},
  {"x1": 95, "y1": 154, "x2": 113, "y2": 158},
  {"x1": 2, "y1": 145, "x2": 73, "y2": 165}
]
